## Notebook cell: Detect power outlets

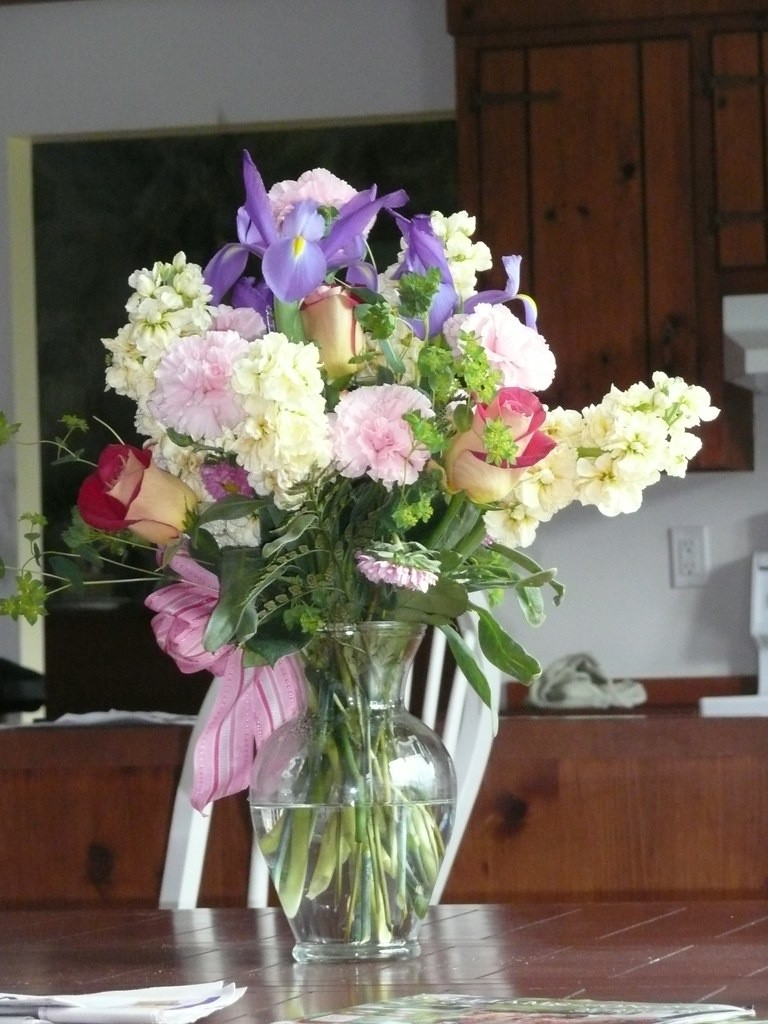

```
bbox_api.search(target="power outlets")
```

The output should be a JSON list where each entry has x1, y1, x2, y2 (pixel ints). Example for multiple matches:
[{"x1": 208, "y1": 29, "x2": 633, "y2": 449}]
[{"x1": 671, "y1": 524, "x2": 712, "y2": 588}]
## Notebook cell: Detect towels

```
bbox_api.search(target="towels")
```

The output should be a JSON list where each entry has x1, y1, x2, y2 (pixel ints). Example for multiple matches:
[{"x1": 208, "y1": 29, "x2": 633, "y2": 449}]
[{"x1": 529, "y1": 650, "x2": 648, "y2": 711}]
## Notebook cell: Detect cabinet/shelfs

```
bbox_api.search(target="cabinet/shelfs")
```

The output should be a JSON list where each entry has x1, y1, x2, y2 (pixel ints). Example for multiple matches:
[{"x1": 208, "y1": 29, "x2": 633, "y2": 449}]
[{"x1": 447, "y1": 1, "x2": 767, "y2": 469}]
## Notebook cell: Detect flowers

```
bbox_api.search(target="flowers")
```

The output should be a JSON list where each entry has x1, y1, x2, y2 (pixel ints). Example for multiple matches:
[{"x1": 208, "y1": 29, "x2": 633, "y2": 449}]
[{"x1": 0, "y1": 149, "x2": 720, "y2": 941}]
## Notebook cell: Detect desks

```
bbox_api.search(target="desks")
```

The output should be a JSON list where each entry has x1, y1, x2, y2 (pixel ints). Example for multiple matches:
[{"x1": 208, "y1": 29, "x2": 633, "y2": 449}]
[{"x1": 0, "y1": 903, "x2": 768, "y2": 1024}]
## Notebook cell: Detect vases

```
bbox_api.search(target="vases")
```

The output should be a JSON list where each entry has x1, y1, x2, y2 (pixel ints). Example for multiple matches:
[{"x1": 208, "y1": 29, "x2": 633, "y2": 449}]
[{"x1": 248, "y1": 622, "x2": 457, "y2": 959}]
